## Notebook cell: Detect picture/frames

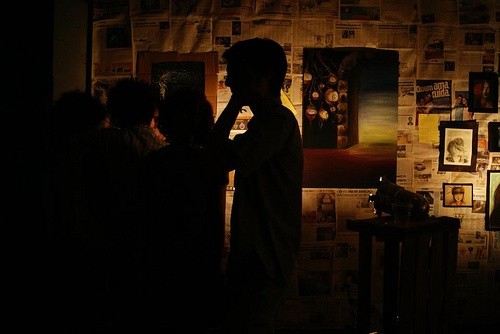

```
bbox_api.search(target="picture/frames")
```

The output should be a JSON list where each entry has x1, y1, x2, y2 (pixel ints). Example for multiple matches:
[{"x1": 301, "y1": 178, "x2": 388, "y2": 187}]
[
  {"x1": 442, "y1": 182, "x2": 474, "y2": 208},
  {"x1": 485, "y1": 170, "x2": 500, "y2": 231},
  {"x1": 469, "y1": 71, "x2": 498, "y2": 112},
  {"x1": 438, "y1": 121, "x2": 479, "y2": 172}
]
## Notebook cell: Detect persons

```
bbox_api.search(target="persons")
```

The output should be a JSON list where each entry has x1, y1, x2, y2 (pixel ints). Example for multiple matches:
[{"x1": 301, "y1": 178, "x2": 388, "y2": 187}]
[
  {"x1": 450, "y1": 187, "x2": 465, "y2": 205},
  {"x1": 416, "y1": 78, "x2": 498, "y2": 121},
  {"x1": 446, "y1": 139, "x2": 467, "y2": 163},
  {"x1": 46, "y1": 38, "x2": 303, "y2": 334}
]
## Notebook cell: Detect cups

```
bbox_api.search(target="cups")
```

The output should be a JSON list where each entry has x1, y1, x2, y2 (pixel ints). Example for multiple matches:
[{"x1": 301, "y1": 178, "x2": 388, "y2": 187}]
[{"x1": 390, "y1": 201, "x2": 413, "y2": 228}]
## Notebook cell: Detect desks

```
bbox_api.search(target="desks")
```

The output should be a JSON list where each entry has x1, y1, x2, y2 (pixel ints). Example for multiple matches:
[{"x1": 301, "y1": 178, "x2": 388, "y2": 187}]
[{"x1": 346, "y1": 212, "x2": 460, "y2": 334}]
